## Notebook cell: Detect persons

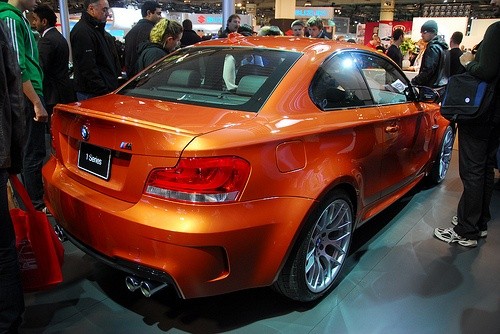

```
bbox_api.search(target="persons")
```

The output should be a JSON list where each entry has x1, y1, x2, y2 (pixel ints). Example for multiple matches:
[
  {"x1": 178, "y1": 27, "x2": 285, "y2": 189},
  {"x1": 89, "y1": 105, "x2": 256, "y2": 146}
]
[
  {"x1": 223, "y1": 26, "x2": 264, "y2": 90},
  {"x1": 0, "y1": 0, "x2": 66, "y2": 243},
  {"x1": 0, "y1": 20, "x2": 25, "y2": 334},
  {"x1": 445, "y1": 31, "x2": 467, "y2": 77},
  {"x1": 410, "y1": 19, "x2": 450, "y2": 104},
  {"x1": 433, "y1": 21, "x2": 500, "y2": 248},
  {"x1": 180, "y1": 19, "x2": 203, "y2": 48},
  {"x1": 219, "y1": 14, "x2": 388, "y2": 54},
  {"x1": 69, "y1": 0, "x2": 121, "y2": 102},
  {"x1": 387, "y1": 29, "x2": 405, "y2": 70},
  {"x1": 124, "y1": 0, "x2": 162, "y2": 81},
  {"x1": 135, "y1": 18, "x2": 183, "y2": 75},
  {"x1": 402, "y1": 38, "x2": 480, "y2": 71},
  {"x1": 33, "y1": 4, "x2": 68, "y2": 217}
]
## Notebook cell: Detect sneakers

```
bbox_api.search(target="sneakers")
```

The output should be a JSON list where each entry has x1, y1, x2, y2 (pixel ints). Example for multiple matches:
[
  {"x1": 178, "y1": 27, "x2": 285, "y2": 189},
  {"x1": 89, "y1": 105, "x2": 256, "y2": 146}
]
[
  {"x1": 434, "y1": 227, "x2": 477, "y2": 247},
  {"x1": 452, "y1": 215, "x2": 488, "y2": 237}
]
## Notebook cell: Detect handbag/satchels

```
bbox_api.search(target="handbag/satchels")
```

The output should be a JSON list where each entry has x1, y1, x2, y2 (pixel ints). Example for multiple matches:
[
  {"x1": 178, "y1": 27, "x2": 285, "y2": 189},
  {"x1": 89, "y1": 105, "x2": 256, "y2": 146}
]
[
  {"x1": 440, "y1": 73, "x2": 493, "y2": 119},
  {"x1": 6, "y1": 176, "x2": 65, "y2": 293}
]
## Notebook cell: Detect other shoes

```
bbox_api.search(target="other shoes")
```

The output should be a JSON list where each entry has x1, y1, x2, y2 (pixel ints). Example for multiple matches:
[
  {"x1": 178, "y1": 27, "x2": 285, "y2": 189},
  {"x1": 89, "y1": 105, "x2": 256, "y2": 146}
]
[
  {"x1": 35, "y1": 203, "x2": 53, "y2": 216},
  {"x1": 52, "y1": 224, "x2": 67, "y2": 242}
]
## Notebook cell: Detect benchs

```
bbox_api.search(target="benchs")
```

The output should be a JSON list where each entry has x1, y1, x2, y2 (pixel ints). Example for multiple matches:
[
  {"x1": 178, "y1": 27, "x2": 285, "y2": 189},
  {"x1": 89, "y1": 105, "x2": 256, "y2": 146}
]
[{"x1": 158, "y1": 69, "x2": 269, "y2": 101}]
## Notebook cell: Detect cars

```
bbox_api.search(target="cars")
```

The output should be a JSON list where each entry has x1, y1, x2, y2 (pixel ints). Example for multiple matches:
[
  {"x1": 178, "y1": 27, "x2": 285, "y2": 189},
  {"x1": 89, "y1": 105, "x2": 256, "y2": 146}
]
[{"x1": 40, "y1": 34, "x2": 458, "y2": 308}]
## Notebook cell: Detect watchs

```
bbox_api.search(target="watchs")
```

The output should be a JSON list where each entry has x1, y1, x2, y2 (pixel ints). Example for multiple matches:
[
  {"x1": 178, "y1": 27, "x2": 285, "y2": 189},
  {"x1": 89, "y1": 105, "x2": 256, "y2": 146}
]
[{"x1": 464, "y1": 61, "x2": 471, "y2": 67}]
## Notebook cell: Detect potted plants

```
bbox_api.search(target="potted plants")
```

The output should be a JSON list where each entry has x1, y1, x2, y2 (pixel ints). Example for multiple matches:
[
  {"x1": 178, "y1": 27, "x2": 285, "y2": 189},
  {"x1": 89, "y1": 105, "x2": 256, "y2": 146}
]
[{"x1": 399, "y1": 37, "x2": 416, "y2": 67}]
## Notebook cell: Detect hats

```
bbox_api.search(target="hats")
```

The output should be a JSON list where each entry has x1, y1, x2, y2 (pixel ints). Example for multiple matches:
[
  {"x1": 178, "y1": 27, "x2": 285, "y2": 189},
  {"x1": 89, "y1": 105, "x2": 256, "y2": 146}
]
[{"x1": 420, "y1": 20, "x2": 438, "y2": 33}]
